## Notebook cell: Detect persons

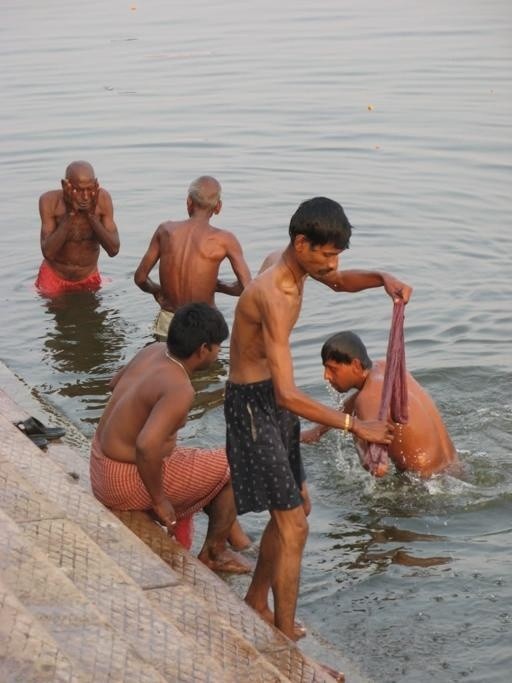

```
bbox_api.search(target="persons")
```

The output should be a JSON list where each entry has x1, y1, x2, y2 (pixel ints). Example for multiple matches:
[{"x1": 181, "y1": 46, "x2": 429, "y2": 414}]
[
  {"x1": 224, "y1": 197, "x2": 413, "y2": 640},
  {"x1": 34, "y1": 161, "x2": 120, "y2": 297},
  {"x1": 90, "y1": 302, "x2": 255, "y2": 573},
  {"x1": 132, "y1": 175, "x2": 252, "y2": 336},
  {"x1": 300, "y1": 331, "x2": 463, "y2": 479}
]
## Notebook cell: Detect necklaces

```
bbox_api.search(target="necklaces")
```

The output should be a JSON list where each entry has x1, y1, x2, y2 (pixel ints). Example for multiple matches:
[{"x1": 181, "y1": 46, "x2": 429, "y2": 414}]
[
  {"x1": 281, "y1": 254, "x2": 302, "y2": 295},
  {"x1": 166, "y1": 350, "x2": 191, "y2": 384}
]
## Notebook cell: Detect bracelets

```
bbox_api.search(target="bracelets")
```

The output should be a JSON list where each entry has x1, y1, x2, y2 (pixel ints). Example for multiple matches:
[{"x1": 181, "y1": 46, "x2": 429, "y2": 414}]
[{"x1": 344, "y1": 414, "x2": 351, "y2": 436}]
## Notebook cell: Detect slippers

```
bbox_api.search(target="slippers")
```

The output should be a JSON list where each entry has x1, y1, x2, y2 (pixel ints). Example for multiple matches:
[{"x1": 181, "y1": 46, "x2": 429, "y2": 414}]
[{"x1": 12, "y1": 416, "x2": 66, "y2": 448}]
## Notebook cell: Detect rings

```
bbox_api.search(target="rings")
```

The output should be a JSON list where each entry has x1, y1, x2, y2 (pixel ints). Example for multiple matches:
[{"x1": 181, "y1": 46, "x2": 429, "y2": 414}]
[{"x1": 172, "y1": 521, "x2": 176, "y2": 525}]
